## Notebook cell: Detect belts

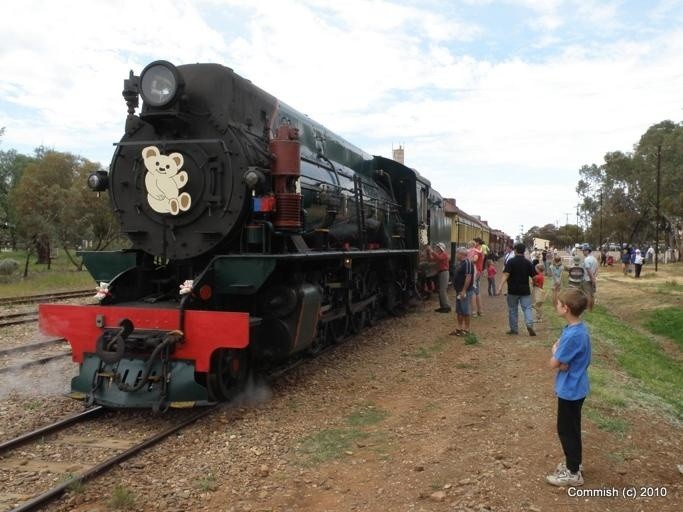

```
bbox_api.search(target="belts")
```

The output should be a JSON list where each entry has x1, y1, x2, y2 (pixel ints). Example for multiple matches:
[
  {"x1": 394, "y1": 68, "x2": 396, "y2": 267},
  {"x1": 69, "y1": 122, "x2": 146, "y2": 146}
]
[{"x1": 439, "y1": 269, "x2": 447, "y2": 272}]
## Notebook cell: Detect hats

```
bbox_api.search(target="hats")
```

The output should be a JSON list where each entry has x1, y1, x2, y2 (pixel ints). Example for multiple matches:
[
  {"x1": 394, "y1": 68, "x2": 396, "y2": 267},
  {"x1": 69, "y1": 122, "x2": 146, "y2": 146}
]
[
  {"x1": 436, "y1": 243, "x2": 446, "y2": 252},
  {"x1": 553, "y1": 256, "x2": 561, "y2": 265},
  {"x1": 581, "y1": 244, "x2": 592, "y2": 251},
  {"x1": 574, "y1": 255, "x2": 580, "y2": 265}
]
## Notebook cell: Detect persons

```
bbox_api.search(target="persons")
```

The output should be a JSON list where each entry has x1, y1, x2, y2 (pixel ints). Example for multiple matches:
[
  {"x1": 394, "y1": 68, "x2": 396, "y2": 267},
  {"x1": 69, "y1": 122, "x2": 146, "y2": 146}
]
[
  {"x1": 417, "y1": 234, "x2": 681, "y2": 337},
  {"x1": 543, "y1": 286, "x2": 592, "y2": 487}
]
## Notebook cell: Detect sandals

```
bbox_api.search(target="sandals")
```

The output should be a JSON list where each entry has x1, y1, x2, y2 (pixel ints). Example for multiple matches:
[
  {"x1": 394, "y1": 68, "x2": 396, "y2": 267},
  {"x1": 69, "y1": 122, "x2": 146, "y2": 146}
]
[
  {"x1": 457, "y1": 330, "x2": 470, "y2": 336},
  {"x1": 449, "y1": 330, "x2": 462, "y2": 335},
  {"x1": 471, "y1": 310, "x2": 484, "y2": 318}
]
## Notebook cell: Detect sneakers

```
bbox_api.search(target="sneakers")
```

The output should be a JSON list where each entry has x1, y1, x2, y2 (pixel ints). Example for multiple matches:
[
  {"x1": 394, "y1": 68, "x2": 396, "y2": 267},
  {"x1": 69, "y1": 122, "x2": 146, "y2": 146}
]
[
  {"x1": 506, "y1": 330, "x2": 518, "y2": 334},
  {"x1": 473, "y1": 284, "x2": 477, "y2": 288},
  {"x1": 546, "y1": 462, "x2": 584, "y2": 487},
  {"x1": 435, "y1": 307, "x2": 452, "y2": 313},
  {"x1": 527, "y1": 324, "x2": 536, "y2": 336}
]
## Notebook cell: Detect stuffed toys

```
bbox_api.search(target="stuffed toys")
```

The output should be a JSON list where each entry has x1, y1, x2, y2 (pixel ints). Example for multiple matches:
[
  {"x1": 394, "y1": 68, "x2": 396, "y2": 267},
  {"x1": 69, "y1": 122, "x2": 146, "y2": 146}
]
[
  {"x1": 91, "y1": 281, "x2": 108, "y2": 301},
  {"x1": 178, "y1": 279, "x2": 193, "y2": 296}
]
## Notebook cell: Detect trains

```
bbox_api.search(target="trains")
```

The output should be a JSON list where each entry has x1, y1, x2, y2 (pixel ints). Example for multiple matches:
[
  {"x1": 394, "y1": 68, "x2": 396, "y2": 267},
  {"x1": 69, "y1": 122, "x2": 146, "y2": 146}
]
[{"x1": 38, "y1": 59, "x2": 511, "y2": 418}]
